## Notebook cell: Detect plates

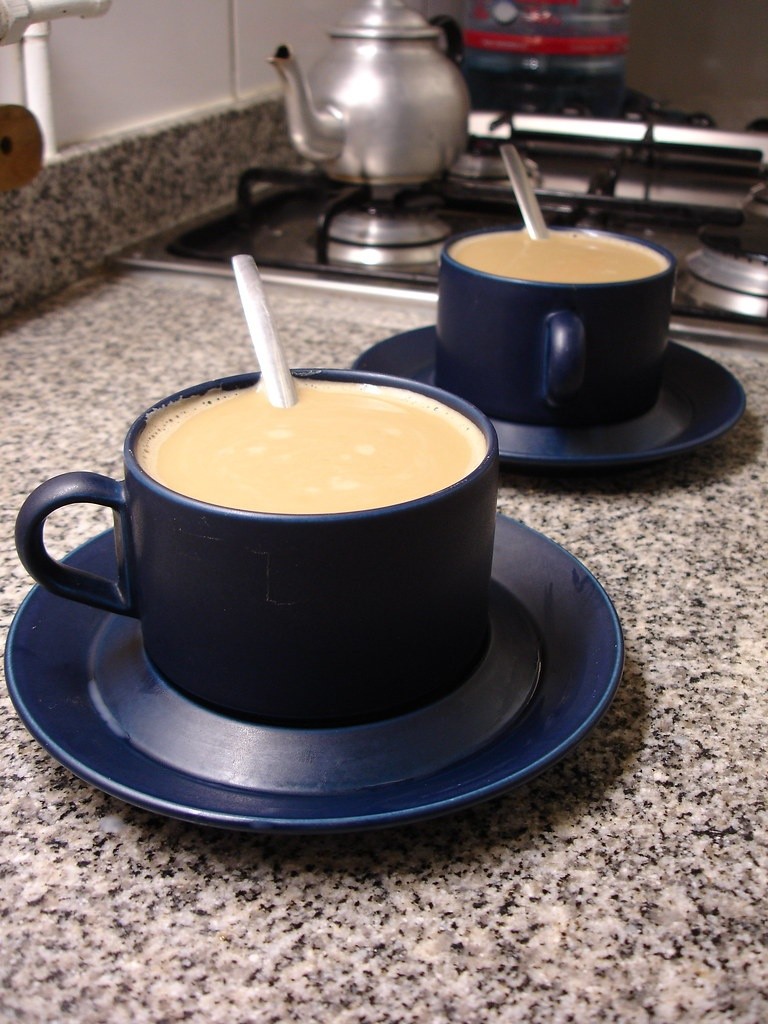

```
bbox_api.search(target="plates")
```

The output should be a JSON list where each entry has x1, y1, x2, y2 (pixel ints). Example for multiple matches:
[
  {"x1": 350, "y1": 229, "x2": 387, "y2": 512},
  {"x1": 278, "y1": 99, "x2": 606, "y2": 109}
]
[
  {"x1": 343, "y1": 324, "x2": 746, "y2": 506},
  {"x1": 2, "y1": 514, "x2": 627, "y2": 837}
]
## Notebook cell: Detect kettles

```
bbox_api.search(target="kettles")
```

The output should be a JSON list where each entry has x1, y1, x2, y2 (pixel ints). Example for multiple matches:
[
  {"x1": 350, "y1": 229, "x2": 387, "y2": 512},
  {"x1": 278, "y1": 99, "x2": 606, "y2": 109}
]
[{"x1": 266, "y1": 1, "x2": 471, "y2": 186}]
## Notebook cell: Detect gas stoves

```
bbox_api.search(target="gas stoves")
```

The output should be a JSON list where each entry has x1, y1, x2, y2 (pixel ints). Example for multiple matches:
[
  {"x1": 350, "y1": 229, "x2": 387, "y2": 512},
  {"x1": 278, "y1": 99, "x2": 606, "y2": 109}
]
[{"x1": 113, "y1": 127, "x2": 768, "y2": 338}]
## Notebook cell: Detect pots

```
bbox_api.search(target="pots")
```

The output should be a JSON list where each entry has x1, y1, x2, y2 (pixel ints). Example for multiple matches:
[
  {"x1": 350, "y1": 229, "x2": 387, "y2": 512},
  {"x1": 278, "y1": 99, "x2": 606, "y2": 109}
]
[{"x1": 449, "y1": 0, "x2": 633, "y2": 120}]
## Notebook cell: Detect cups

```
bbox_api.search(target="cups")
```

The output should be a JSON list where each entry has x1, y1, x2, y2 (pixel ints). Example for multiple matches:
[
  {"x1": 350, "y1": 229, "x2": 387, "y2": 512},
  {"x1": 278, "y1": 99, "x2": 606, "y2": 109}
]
[
  {"x1": 432, "y1": 226, "x2": 677, "y2": 431},
  {"x1": 14, "y1": 368, "x2": 501, "y2": 728}
]
